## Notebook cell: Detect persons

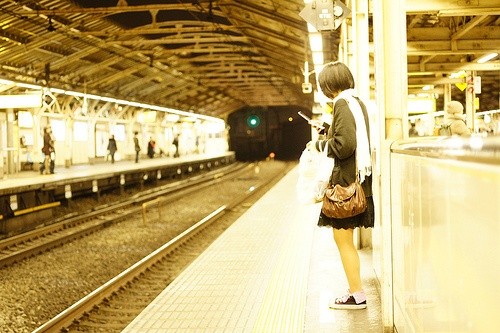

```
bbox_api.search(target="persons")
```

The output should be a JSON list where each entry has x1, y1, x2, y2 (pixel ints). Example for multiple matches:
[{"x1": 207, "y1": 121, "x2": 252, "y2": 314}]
[
  {"x1": 410, "y1": 124, "x2": 419, "y2": 138},
  {"x1": 40, "y1": 127, "x2": 56, "y2": 175},
  {"x1": 147, "y1": 137, "x2": 156, "y2": 160},
  {"x1": 172, "y1": 134, "x2": 181, "y2": 158},
  {"x1": 106, "y1": 135, "x2": 118, "y2": 166},
  {"x1": 441, "y1": 101, "x2": 471, "y2": 137},
  {"x1": 306, "y1": 61, "x2": 376, "y2": 311},
  {"x1": 133, "y1": 131, "x2": 142, "y2": 163}
]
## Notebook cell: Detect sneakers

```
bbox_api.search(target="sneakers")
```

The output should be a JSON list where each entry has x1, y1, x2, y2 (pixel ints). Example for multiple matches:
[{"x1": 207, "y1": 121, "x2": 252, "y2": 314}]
[{"x1": 329, "y1": 288, "x2": 367, "y2": 309}]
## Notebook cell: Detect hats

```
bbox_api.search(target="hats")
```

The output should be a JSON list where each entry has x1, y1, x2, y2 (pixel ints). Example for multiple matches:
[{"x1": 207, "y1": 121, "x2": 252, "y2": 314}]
[{"x1": 447, "y1": 101, "x2": 463, "y2": 114}]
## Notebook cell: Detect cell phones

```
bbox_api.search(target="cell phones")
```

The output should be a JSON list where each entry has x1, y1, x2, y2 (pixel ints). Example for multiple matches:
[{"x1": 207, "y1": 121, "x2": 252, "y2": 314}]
[{"x1": 298, "y1": 111, "x2": 327, "y2": 130}]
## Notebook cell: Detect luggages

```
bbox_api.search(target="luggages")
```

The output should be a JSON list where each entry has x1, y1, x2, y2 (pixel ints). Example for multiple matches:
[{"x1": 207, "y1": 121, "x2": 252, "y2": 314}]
[{"x1": 40, "y1": 152, "x2": 55, "y2": 174}]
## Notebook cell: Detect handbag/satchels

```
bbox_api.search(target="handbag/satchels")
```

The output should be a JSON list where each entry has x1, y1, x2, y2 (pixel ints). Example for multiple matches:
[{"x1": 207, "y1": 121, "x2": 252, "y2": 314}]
[
  {"x1": 321, "y1": 180, "x2": 366, "y2": 219},
  {"x1": 298, "y1": 138, "x2": 335, "y2": 207}
]
